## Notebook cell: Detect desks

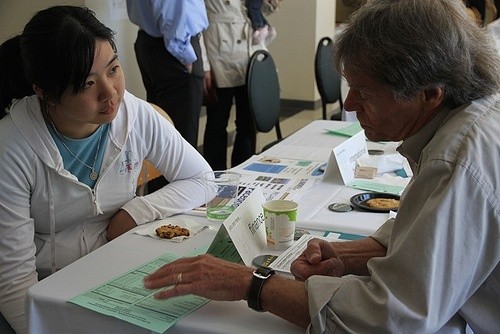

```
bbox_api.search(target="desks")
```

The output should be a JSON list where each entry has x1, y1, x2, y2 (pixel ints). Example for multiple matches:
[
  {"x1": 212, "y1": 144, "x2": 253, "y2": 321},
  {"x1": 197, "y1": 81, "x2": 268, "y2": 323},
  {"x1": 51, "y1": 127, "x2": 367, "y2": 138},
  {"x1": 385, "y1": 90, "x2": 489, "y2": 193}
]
[{"x1": 25, "y1": 119, "x2": 470, "y2": 334}]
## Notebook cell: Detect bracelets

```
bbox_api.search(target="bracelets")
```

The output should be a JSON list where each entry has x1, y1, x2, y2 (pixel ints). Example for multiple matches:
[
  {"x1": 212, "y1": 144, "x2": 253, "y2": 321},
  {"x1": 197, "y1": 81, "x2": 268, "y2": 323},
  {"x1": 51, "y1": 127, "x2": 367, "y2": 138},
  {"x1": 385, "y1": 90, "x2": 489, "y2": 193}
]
[{"x1": 247, "y1": 267, "x2": 277, "y2": 312}]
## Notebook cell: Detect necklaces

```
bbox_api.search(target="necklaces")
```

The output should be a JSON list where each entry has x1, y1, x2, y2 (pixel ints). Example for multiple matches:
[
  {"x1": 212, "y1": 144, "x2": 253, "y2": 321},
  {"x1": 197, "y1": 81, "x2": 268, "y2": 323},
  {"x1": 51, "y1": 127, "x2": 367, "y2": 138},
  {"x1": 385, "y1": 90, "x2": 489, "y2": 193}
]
[{"x1": 44, "y1": 103, "x2": 104, "y2": 180}]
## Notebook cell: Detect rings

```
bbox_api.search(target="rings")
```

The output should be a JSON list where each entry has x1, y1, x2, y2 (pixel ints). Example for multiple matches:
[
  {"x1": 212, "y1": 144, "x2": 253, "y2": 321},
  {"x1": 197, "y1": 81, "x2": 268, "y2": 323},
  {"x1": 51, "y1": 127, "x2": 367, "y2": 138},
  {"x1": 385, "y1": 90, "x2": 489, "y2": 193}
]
[{"x1": 176, "y1": 272, "x2": 182, "y2": 284}]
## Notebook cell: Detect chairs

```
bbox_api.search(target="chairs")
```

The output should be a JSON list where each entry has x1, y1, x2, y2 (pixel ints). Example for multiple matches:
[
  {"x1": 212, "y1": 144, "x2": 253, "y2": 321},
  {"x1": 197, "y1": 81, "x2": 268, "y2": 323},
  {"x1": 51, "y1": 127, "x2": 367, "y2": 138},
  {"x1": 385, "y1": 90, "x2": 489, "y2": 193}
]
[
  {"x1": 314, "y1": 37, "x2": 344, "y2": 121},
  {"x1": 137, "y1": 102, "x2": 176, "y2": 187},
  {"x1": 245, "y1": 49, "x2": 283, "y2": 153}
]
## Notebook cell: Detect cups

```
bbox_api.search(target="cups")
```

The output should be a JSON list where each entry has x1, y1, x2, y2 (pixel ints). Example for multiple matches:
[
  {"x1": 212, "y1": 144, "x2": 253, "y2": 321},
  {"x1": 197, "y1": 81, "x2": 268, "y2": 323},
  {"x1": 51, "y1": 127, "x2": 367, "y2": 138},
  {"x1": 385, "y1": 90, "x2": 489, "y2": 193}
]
[
  {"x1": 201, "y1": 171, "x2": 241, "y2": 222},
  {"x1": 261, "y1": 199, "x2": 299, "y2": 251}
]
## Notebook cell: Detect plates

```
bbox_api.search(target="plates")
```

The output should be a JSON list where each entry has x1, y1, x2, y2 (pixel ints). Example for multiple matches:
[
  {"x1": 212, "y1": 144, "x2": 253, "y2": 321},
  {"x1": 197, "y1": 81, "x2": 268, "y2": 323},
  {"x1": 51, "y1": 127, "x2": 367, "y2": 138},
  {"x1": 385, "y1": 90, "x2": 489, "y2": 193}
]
[{"x1": 350, "y1": 193, "x2": 401, "y2": 213}]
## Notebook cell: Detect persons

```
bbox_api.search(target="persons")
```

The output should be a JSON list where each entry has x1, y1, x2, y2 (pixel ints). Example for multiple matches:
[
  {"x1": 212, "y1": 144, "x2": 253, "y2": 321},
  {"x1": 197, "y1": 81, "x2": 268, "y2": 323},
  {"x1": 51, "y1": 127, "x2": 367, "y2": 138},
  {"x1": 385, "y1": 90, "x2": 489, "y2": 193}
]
[
  {"x1": 126, "y1": 0, "x2": 281, "y2": 194},
  {"x1": 0, "y1": 6, "x2": 216, "y2": 334},
  {"x1": 146, "y1": 0, "x2": 500, "y2": 334}
]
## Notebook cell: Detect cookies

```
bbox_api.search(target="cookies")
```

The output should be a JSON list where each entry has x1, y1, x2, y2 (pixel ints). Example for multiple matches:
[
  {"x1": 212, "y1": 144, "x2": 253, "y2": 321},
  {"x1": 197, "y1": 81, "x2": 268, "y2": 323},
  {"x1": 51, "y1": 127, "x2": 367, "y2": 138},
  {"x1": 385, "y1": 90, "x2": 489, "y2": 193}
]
[
  {"x1": 156, "y1": 225, "x2": 189, "y2": 239},
  {"x1": 366, "y1": 197, "x2": 400, "y2": 208}
]
